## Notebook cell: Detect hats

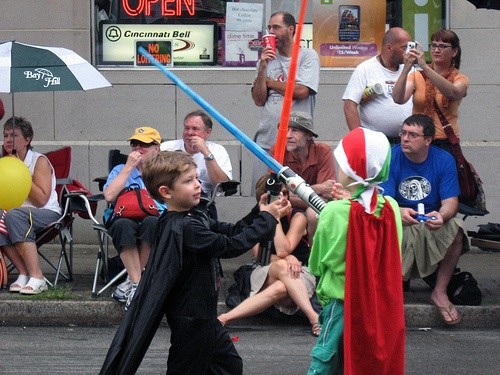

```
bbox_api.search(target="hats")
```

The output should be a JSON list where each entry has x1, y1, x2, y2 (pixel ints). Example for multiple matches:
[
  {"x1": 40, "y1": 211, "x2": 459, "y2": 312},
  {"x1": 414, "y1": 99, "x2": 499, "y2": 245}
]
[
  {"x1": 278, "y1": 112, "x2": 319, "y2": 138},
  {"x1": 128, "y1": 127, "x2": 161, "y2": 143}
]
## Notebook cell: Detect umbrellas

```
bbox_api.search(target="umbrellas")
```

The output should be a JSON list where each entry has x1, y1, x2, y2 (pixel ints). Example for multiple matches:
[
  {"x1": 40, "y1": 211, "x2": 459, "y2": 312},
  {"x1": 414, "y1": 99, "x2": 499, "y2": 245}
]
[{"x1": 0, "y1": 39, "x2": 113, "y2": 156}]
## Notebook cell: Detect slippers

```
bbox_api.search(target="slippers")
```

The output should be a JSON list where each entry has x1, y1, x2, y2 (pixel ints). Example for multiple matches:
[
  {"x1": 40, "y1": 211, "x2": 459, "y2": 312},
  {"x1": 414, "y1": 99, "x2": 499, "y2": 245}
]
[{"x1": 436, "y1": 304, "x2": 462, "y2": 325}]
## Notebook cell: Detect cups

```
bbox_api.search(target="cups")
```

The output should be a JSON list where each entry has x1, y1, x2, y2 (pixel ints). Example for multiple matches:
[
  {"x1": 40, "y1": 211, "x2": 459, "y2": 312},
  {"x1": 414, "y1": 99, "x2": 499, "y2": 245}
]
[{"x1": 262, "y1": 34, "x2": 276, "y2": 60}]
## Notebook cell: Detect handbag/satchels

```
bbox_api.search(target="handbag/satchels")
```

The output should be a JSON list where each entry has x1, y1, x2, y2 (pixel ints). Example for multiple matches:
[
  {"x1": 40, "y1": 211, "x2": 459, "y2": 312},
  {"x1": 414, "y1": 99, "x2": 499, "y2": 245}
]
[
  {"x1": 226, "y1": 264, "x2": 254, "y2": 308},
  {"x1": 114, "y1": 190, "x2": 160, "y2": 223},
  {"x1": 101, "y1": 255, "x2": 128, "y2": 288},
  {"x1": 458, "y1": 162, "x2": 489, "y2": 216}
]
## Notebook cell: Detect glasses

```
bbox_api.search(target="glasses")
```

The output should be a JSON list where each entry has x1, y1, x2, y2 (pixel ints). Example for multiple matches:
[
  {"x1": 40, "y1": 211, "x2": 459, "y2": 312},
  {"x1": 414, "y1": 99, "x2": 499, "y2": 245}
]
[
  {"x1": 130, "y1": 140, "x2": 157, "y2": 147},
  {"x1": 429, "y1": 44, "x2": 456, "y2": 50},
  {"x1": 399, "y1": 131, "x2": 431, "y2": 139}
]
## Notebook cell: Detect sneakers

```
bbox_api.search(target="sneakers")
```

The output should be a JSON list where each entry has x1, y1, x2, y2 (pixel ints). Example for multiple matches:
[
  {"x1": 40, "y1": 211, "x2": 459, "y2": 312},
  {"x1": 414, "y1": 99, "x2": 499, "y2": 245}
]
[{"x1": 113, "y1": 280, "x2": 138, "y2": 310}]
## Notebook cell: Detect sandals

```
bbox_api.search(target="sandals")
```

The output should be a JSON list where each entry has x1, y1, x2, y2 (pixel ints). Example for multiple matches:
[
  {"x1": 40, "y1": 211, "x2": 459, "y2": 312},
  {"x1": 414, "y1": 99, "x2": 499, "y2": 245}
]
[
  {"x1": 312, "y1": 323, "x2": 322, "y2": 336},
  {"x1": 9, "y1": 274, "x2": 48, "y2": 294}
]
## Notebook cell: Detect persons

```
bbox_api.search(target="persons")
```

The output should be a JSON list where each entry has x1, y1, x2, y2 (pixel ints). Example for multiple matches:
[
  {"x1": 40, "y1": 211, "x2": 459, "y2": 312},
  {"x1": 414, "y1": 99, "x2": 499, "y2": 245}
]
[
  {"x1": 268, "y1": 111, "x2": 337, "y2": 250},
  {"x1": 103, "y1": 127, "x2": 167, "y2": 311},
  {"x1": 217, "y1": 173, "x2": 322, "y2": 337},
  {"x1": 307, "y1": 127, "x2": 405, "y2": 375},
  {"x1": 392, "y1": 30, "x2": 471, "y2": 155},
  {"x1": 99, "y1": 150, "x2": 283, "y2": 375},
  {"x1": 251, "y1": 11, "x2": 320, "y2": 197},
  {"x1": 342, "y1": 27, "x2": 423, "y2": 149},
  {"x1": 159, "y1": 111, "x2": 233, "y2": 221},
  {"x1": 377, "y1": 114, "x2": 471, "y2": 324},
  {"x1": 0, "y1": 116, "x2": 62, "y2": 294}
]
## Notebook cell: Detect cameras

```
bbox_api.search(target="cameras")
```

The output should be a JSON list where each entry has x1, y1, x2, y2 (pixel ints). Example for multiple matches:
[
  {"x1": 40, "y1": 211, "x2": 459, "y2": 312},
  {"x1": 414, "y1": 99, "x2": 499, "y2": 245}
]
[
  {"x1": 416, "y1": 214, "x2": 436, "y2": 223},
  {"x1": 406, "y1": 42, "x2": 417, "y2": 55}
]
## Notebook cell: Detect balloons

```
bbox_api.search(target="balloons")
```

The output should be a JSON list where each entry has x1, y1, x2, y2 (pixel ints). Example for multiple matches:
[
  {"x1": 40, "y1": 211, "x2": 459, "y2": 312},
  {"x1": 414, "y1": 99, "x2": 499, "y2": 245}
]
[{"x1": 0, "y1": 156, "x2": 32, "y2": 211}]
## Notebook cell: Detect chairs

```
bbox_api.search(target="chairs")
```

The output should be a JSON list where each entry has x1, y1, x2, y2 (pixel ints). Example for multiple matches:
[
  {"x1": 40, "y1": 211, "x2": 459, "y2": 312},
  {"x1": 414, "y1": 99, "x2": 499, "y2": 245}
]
[
  {"x1": 6, "y1": 147, "x2": 97, "y2": 287},
  {"x1": 64, "y1": 146, "x2": 240, "y2": 297}
]
